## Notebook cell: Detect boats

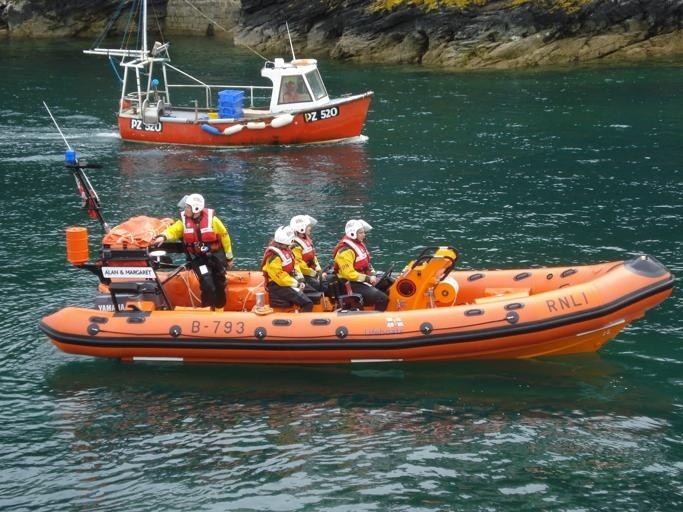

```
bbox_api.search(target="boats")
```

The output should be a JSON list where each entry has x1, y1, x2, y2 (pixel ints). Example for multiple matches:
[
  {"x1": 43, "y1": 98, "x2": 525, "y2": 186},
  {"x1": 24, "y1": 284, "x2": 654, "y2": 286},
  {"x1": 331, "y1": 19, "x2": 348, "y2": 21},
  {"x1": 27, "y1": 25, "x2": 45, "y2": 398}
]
[
  {"x1": 39, "y1": 100, "x2": 676, "y2": 365},
  {"x1": 81, "y1": 0, "x2": 376, "y2": 145}
]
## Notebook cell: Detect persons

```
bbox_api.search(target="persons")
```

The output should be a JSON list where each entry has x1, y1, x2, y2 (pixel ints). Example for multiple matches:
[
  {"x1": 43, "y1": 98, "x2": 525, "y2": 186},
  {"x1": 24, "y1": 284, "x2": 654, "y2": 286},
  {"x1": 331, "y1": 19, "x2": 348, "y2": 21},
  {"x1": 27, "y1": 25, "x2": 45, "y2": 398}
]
[
  {"x1": 290, "y1": 214, "x2": 334, "y2": 296},
  {"x1": 283, "y1": 81, "x2": 297, "y2": 103},
  {"x1": 262, "y1": 226, "x2": 313, "y2": 312},
  {"x1": 148, "y1": 194, "x2": 231, "y2": 311},
  {"x1": 333, "y1": 220, "x2": 389, "y2": 313}
]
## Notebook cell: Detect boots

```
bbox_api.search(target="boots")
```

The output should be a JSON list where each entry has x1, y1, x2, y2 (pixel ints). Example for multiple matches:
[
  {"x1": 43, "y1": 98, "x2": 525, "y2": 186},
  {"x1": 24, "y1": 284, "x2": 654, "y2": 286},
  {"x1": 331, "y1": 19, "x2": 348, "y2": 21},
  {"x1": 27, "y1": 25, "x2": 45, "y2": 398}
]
[{"x1": 215, "y1": 307, "x2": 223, "y2": 311}]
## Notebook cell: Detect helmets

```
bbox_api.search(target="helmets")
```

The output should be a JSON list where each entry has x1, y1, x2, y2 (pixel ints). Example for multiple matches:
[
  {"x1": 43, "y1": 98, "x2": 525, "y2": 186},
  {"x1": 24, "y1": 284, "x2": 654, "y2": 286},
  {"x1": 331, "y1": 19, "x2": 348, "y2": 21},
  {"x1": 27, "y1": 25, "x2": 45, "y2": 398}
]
[
  {"x1": 345, "y1": 219, "x2": 372, "y2": 239},
  {"x1": 290, "y1": 215, "x2": 317, "y2": 233},
  {"x1": 274, "y1": 225, "x2": 295, "y2": 245},
  {"x1": 178, "y1": 194, "x2": 205, "y2": 214}
]
